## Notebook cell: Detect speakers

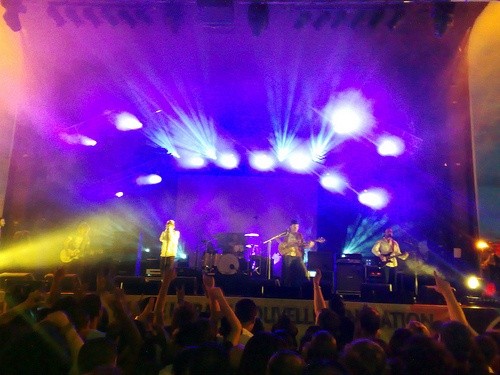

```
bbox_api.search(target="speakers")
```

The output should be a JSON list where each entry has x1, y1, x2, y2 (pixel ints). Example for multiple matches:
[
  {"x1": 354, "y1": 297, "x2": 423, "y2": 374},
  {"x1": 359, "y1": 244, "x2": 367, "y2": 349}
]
[
  {"x1": 0, "y1": 272, "x2": 34, "y2": 289},
  {"x1": 44, "y1": 273, "x2": 81, "y2": 293},
  {"x1": 307, "y1": 251, "x2": 365, "y2": 292}
]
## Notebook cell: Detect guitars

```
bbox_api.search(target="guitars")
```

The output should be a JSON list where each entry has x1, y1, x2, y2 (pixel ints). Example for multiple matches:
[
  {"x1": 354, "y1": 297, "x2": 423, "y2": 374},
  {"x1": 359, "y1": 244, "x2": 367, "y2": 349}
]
[
  {"x1": 277, "y1": 236, "x2": 327, "y2": 256},
  {"x1": 371, "y1": 249, "x2": 415, "y2": 267},
  {"x1": 59, "y1": 248, "x2": 104, "y2": 263}
]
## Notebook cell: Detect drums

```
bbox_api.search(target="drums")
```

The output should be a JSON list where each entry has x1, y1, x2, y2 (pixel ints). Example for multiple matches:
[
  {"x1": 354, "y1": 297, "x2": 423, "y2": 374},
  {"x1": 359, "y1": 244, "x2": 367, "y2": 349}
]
[
  {"x1": 201, "y1": 252, "x2": 221, "y2": 271},
  {"x1": 216, "y1": 252, "x2": 242, "y2": 276}
]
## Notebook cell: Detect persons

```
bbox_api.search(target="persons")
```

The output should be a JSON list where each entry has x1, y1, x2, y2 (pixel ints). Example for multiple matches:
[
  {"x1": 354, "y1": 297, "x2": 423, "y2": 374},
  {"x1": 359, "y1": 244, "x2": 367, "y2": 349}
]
[
  {"x1": 0, "y1": 267, "x2": 500, "y2": 375},
  {"x1": 61, "y1": 222, "x2": 96, "y2": 290},
  {"x1": 159, "y1": 220, "x2": 179, "y2": 274},
  {"x1": 480, "y1": 243, "x2": 500, "y2": 295},
  {"x1": 371, "y1": 228, "x2": 408, "y2": 301},
  {"x1": 275, "y1": 221, "x2": 314, "y2": 271}
]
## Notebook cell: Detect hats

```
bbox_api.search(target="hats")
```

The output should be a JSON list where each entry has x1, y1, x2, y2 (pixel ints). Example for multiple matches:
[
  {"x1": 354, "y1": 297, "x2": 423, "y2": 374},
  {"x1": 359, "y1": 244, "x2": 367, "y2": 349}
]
[{"x1": 289, "y1": 220, "x2": 299, "y2": 225}]
